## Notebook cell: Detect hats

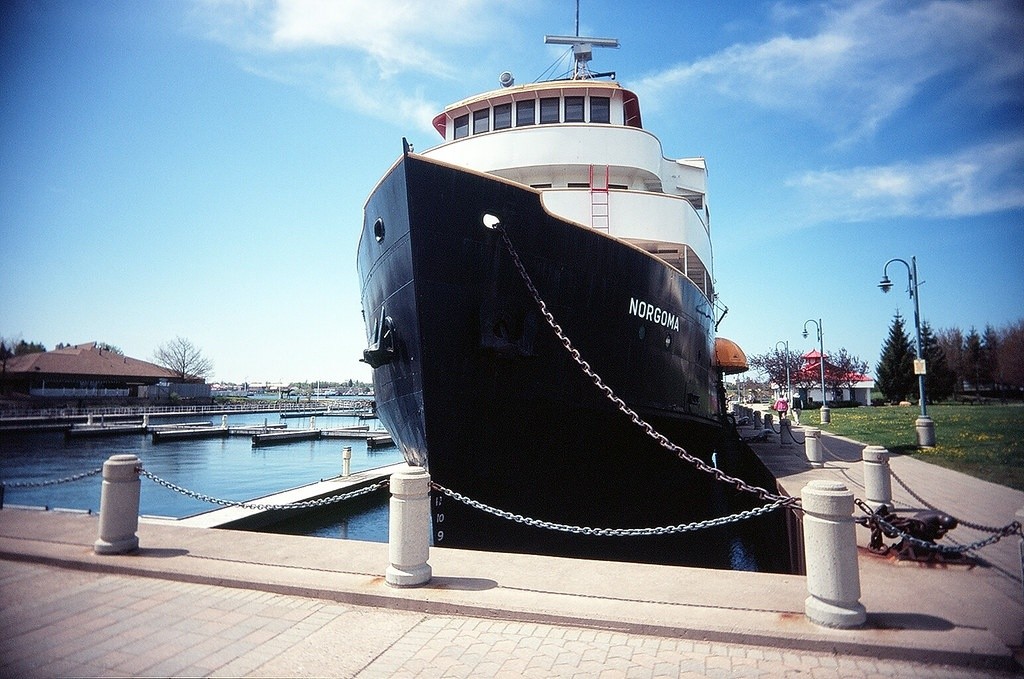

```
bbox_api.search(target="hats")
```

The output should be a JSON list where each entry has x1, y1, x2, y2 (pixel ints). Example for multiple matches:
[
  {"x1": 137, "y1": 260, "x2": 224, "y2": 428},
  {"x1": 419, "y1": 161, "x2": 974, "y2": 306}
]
[{"x1": 792, "y1": 393, "x2": 801, "y2": 399}]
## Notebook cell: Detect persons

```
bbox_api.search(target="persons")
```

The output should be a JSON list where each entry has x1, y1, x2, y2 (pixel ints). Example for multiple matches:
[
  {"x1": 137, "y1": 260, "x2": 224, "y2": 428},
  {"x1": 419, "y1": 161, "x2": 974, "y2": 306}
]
[
  {"x1": 774, "y1": 395, "x2": 789, "y2": 423},
  {"x1": 719, "y1": 384, "x2": 726, "y2": 411},
  {"x1": 791, "y1": 393, "x2": 803, "y2": 425}
]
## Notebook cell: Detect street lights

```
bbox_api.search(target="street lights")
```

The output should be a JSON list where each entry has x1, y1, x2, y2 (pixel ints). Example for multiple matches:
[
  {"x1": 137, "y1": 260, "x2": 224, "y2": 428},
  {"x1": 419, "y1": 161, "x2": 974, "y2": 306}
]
[
  {"x1": 877, "y1": 256, "x2": 937, "y2": 446},
  {"x1": 773, "y1": 340, "x2": 790, "y2": 406},
  {"x1": 802, "y1": 318, "x2": 832, "y2": 424}
]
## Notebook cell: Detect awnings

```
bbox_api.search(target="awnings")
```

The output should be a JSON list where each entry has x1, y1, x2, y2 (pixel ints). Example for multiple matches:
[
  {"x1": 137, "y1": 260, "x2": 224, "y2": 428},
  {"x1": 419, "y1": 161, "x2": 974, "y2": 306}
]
[{"x1": 716, "y1": 337, "x2": 748, "y2": 375}]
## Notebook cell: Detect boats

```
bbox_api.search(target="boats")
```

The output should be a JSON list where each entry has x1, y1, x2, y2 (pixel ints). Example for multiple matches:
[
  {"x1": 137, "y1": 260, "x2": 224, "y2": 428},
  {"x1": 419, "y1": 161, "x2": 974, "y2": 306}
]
[
  {"x1": 355, "y1": 0, "x2": 751, "y2": 541},
  {"x1": 714, "y1": 336, "x2": 750, "y2": 375}
]
